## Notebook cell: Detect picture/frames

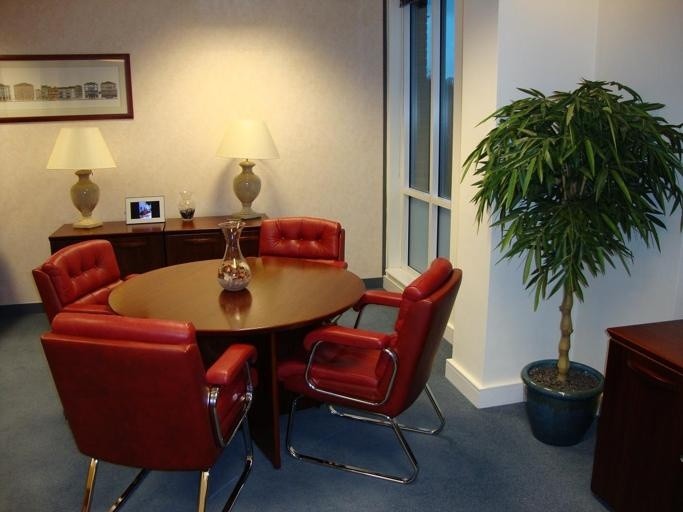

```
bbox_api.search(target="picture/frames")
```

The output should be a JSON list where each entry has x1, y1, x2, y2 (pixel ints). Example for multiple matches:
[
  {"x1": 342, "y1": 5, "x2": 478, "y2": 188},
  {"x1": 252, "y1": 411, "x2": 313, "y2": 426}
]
[
  {"x1": 125, "y1": 196, "x2": 165, "y2": 225},
  {"x1": 0, "y1": 53, "x2": 135, "y2": 123}
]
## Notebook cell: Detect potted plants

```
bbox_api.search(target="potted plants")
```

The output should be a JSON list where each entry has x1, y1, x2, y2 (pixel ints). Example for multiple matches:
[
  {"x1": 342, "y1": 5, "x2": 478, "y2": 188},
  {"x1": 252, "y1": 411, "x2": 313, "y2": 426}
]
[{"x1": 457, "y1": 76, "x2": 683, "y2": 446}]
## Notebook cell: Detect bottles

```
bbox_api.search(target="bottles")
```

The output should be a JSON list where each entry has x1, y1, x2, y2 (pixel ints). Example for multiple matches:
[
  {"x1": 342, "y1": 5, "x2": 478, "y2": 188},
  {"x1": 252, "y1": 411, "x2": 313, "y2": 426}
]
[
  {"x1": 177, "y1": 190, "x2": 196, "y2": 222},
  {"x1": 218, "y1": 220, "x2": 253, "y2": 293}
]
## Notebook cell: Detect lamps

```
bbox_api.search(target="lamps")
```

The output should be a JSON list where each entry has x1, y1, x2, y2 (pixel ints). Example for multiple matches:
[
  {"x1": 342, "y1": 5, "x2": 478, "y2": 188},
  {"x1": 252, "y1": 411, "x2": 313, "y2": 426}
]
[
  {"x1": 46, "y1": 127, "x2": 118, "y2": 227},
  {"x1": 221, "y1": 118, "x2": 280, "y2": 220}
]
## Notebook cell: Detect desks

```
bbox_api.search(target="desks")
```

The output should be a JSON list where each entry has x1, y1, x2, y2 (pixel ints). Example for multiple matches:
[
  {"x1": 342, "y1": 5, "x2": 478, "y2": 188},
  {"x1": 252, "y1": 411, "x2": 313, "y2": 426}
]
[
  {"x1": 109, "y1": 253, "x2": 365, "y2": 471},
  {"x1": 46, "y1": 208, "x2": 268, "y2": 278}
]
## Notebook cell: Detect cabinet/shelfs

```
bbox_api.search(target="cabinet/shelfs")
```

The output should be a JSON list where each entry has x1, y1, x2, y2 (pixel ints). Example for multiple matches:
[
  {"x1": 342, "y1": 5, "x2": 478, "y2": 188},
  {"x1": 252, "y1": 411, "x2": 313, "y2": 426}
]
[
  {"x1": 163, "y1": 215, "x2": 271, "y2": 266},
  {"x1": 590, "y1": 319, "x2": 683, "y2": 512}
]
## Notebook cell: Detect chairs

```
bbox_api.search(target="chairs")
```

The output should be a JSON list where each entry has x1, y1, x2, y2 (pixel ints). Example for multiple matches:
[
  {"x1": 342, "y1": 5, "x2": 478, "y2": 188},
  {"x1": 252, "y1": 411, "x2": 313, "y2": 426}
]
[
  {"x1": 30, "y1": 238, "x2": 142, "y2": 324},
  {"x1": 258, "y1": 218, "x2": 352, "y2": 331},
  {"x1": 276, "y1": 255, "x2": 463, "y2": 485},
  {"x1": 39, "y1": 310, "x2": 263, "y2": 511}
]
